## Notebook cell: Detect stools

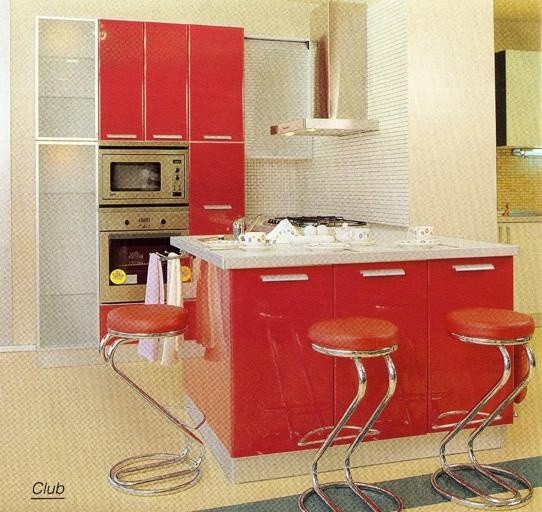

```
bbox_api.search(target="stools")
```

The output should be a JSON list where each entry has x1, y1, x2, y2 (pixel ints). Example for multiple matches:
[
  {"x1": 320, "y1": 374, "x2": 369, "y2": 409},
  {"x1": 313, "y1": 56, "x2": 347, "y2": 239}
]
[
  {"x1": 96, "y1": 300, "x2": 211, "y2": 496},
  {"x1": 297, "y1": 314, "x2": 403, "y2": 511},
  {"x1": 429, "y1": 304, "x2": 537, "y2": 509}
]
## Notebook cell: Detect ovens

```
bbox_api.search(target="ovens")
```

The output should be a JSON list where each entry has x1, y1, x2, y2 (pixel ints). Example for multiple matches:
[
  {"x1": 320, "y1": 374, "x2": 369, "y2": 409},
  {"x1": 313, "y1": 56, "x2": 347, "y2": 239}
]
[{"x1": 98, "y1": 207, "x2": 197, "y2": 305}]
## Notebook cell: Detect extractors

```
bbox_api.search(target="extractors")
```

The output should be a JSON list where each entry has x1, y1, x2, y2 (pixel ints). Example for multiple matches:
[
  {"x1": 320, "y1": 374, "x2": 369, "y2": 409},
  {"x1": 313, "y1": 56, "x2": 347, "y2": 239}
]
[{"x1": 268, "y1": 1, "x2": 378, "y2": 139}]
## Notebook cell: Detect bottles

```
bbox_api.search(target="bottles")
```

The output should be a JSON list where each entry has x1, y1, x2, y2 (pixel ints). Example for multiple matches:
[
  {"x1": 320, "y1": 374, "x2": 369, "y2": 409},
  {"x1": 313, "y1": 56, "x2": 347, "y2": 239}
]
[{"x1": 233, "y1": 220, "x2": 245, "y2": 242}]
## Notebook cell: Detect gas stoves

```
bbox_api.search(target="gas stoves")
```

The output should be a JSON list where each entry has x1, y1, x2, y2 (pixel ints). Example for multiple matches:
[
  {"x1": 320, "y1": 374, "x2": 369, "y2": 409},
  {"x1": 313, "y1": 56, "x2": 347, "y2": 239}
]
[{"x1": 262, "y1": 214, "x2": 371, "y2": 231}]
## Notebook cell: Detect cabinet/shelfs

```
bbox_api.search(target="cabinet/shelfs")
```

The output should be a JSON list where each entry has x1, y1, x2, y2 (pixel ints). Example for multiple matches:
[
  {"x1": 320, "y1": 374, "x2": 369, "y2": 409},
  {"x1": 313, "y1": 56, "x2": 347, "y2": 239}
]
[
  {"x1": 241, "y1": 35, "x2": 316, "y2": 161},
  {"x1": 497, "y1": 220, "x2": 541, "y2": 317},
  {"x1": 36, "y1": 143, "x2": 101, "y2": 354},
  {"x1": 495, "y1": 49, "x2": 541, "y2": 150},
  {"x1": 97, "y1": 19, "x2": 188, "y2": 143},
  {"x1": 190, "y1": 140, "x2": 247, "y2": 235},
  {"x1": 33, "y1": 16, "x2": 99, "y2": 139},
  {"x1": 188, "y1": 25, "x2": 245, "y2": 143}
]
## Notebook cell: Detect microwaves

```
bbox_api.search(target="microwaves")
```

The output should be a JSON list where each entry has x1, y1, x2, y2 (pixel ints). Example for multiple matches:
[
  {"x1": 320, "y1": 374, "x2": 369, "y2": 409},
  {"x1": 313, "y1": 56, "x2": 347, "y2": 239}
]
[{"x1": 100, "y1": 149, "x2": 189, "y2": 205}]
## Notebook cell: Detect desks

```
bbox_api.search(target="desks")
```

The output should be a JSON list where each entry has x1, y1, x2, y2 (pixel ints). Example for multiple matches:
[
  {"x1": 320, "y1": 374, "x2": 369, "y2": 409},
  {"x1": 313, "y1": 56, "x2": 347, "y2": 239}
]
[{"x1": 169, "y1": 226, "x2": 518, "y2": 481}]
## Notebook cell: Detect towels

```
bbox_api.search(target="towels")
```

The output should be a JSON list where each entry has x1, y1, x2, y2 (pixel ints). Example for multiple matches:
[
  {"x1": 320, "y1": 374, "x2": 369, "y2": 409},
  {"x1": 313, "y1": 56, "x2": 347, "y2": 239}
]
[
  {"x1": 138, "y1": 250, "x2": 165, "y2": 360},
  {"x1": 159, "y1": 251, "x2": 189, "y2": 364},
  {"x1": 196, "y1": 263, "x2": 228, "y2": 368}
]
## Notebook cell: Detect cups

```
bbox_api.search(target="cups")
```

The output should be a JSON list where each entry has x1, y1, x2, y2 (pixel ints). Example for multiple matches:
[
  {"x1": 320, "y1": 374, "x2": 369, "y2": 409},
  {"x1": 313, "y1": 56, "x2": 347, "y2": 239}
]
[
  {"x1": 408, "y1": 225, "x2": 433, "y2": 239},
  {"x1": 239, "y1": 232, "x2": 268, "y2": 247},
  {"x1": 275, "y1": 222, "x2": 376, "y2": 250}
]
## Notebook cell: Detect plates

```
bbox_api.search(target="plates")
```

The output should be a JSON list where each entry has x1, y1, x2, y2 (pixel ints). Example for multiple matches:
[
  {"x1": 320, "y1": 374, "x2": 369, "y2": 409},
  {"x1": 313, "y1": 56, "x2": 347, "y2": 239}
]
[
  {"x1": 241, "y1": 246, "x2": 273, "y2": 252},
  {"x1": 394, "y1": 239, "x2": 439, "y2": 248},
  {"x1": 205, "y1": 240, "x2": 237, "y2": 249}
]
